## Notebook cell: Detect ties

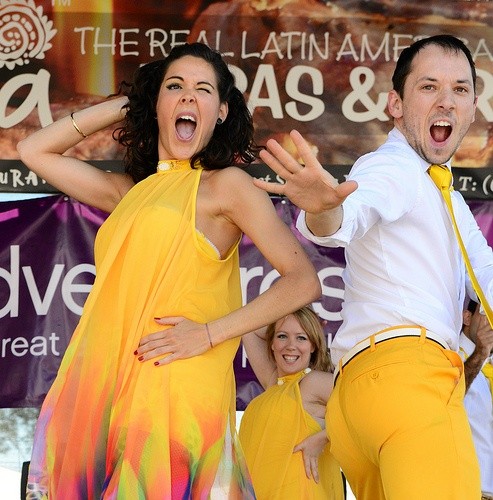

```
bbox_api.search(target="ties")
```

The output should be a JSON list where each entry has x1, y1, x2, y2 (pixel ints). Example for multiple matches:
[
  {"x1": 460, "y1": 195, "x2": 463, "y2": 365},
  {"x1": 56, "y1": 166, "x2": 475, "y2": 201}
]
[
  {"x1": 427, "y1": 164, "x2": 493, "y2": 330},
  {"x1": 459, "y1": 347, "x2": 493, "y2": 395}
]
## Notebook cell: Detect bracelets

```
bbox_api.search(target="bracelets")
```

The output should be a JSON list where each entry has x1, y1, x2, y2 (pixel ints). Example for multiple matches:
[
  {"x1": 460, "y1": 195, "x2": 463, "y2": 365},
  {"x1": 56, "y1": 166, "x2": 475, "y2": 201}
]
[
  {"x1": 71, "y1": 112, "x2": 88, "y2": 138},
  {"x1": 205, "y1": 322, "x2": 214, "y2": 349}
]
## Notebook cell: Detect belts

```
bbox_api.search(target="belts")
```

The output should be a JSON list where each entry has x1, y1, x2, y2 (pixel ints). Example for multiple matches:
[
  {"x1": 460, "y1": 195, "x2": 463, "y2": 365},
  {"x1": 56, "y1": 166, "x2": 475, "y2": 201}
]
[{"x1": 333, "y1": 328, "x2": 452, "y2": 387}]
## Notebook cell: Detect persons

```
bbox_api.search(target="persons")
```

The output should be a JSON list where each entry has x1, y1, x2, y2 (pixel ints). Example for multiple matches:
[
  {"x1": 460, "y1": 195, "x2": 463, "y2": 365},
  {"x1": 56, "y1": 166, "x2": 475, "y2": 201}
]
[
  {"x1": 238, "y1": 304, "x2": 345, "y2": 499},
  {"x1": 16, "y1": 42, "x2": 323, "y2": 500},
  {"x1": 251, "y1": 35, "x2": 493, "y2": 500},
  {"x1": 459, "y1": 297, "x2": 493, "y2": 500}
]
